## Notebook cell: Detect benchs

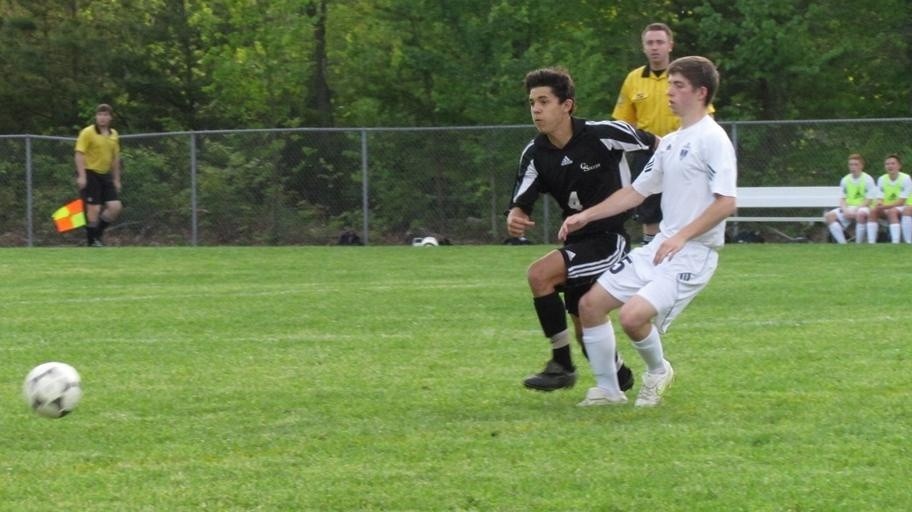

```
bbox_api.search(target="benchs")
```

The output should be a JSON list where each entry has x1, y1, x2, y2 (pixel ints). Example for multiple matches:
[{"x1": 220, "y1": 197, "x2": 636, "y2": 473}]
[{"x1": 727, "y1": 186, "x2": 856, "y2": 243}]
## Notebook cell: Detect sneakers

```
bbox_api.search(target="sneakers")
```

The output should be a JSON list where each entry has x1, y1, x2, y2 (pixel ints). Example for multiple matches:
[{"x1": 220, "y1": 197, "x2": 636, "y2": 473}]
[
  {"x1": 617, "y1": 363, "x2": 634, "y2": 391},
  {"x1": 634, "y1": 359, "x2": 674, "y2": 408},
  {"x1": 523, "y1": 360, "x2": 576, "y2": 391},
  {"x1": 576, "y1": 387, "x2": 628, "y2": 407}
]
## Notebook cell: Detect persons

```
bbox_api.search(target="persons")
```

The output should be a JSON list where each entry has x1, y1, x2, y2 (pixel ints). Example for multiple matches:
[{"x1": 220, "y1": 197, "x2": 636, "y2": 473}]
[
  {"x1": 900, "y1": 205, "x2": 912, "y2": 244},
  {"x1": 866, "y1": 153, "x2": 912, "y2": 244},
  {"x1": 823, "y1": 152, "x2": 875, "y2": 244},
  {"x1": 505, "y1": 65, "x2": 661, "y2": 393},
  {"x1": 71, "y1": 102, "x2": 123, "y2": 247},
  {"x1": 609, "y1": 22, "x2": 716, "y2": 247},
  {"x1": 574, "y1": 56, "x2": 739, "y2": 406}
]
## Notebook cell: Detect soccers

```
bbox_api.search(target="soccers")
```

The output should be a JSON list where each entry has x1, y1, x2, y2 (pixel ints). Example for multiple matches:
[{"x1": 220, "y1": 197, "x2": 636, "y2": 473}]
[{"x1": 25, "y1": 363, "x2": 81, "y2": 417}]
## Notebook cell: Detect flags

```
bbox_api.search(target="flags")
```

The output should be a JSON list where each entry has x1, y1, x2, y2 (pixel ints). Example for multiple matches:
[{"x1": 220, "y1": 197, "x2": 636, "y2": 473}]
[{"x1": 50, "y1": 198, "x2": 87, "y2": 233}]
